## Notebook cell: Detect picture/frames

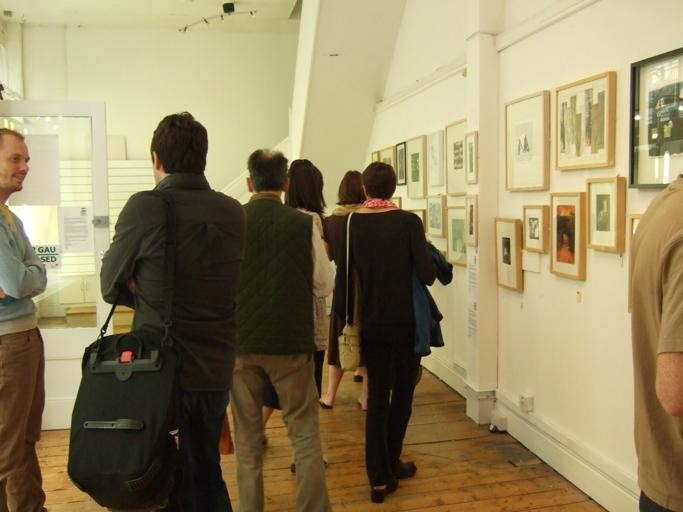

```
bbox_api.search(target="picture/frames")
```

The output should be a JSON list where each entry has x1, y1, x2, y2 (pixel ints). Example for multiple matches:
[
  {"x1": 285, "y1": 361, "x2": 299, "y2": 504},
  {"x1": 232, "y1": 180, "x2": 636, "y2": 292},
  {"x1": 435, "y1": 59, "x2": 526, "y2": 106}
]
[
  {"x1": 409, "y1": 209, "x2": 426, "y2": 232},
  {"x1": 585, "y1": 177, "x2": 626, "y2": 254},
  {"x1": 396, "y1": 142, "x2": 406, "y2": 185},
  {"x1": 522, "y1": 205, "x2": 550, "y2": 254},
  {"x1": 379, "y1": 146, "x2": 395, "y2": 172},
  {"x1": 503, "y1": 91, "x2": 550, "y2": 192},
  {"x1": 549, "y1": 193, "x2": 586, "y2": 281},
  {"x1": 444, "y1": 118, "x2": 467, "y2": 196},
  {"x1": 406, "y1": 135, "x2": 426, "y2": 199},
  {"x1": 393, "y1": 197, "x2": 402, "y2": 208},
  {"x1": 371, "y1": 151, "x2": 379, "y2": 163},
  {"x1": 494, "y1": 217, "x2": 522, "y2": 292},
  {"x1": 446, "y1": 206, "x2": 467, "y2": 268},
  {"x1": 464, "y1": 130, "x2": 478, "y2": 184},
  {"x1": 464, "y1": 195, "x2": 478, "y2": 247},
  {"x1": 628, "y1": 46, "x2": 683, "y2": 190},
  {"x1": 628, "y1": 213, "x2": 643, "y2": 312},
  {"x1": 426, "y1": 130, "x2": 443, "y2": 187},
  {"x1": 426, "y1": 194, "x2": 447, "y2": 238},
  {"x1": 554, "y1": 71, "x2": 617, "y2": 171}
]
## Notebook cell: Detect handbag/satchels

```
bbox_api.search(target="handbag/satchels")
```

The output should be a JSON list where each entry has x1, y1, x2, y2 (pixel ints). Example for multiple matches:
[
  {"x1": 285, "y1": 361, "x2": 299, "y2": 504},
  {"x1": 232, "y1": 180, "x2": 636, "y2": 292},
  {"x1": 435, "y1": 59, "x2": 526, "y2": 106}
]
[
  {"x1": 338, "y1": 213, "x2": 361, "y2": 373},
  {"x1": 67, "y1": 191, "x2": 194, "y2": 512}
]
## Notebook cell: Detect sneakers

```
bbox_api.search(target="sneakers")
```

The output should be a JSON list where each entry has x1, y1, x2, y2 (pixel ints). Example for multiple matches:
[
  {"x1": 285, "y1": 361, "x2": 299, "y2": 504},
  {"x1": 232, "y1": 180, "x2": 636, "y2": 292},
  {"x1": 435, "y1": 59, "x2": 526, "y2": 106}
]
[
  {"x1": 370, "y1": 478, "x2": 398, "y2": 504},
  {"x1": 392, "y1": 459, "x2": 417, "y2": 479}
]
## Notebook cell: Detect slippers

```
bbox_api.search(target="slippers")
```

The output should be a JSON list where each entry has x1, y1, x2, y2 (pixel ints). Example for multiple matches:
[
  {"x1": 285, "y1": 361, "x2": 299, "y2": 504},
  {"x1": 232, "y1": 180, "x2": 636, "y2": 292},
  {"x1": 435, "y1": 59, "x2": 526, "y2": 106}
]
[
  {"x1": 319, "y1": 398, "x2": 333, "y2": 411},
  {"x1": 357, "y1": 399, "x2": 369, "y2": 413}
]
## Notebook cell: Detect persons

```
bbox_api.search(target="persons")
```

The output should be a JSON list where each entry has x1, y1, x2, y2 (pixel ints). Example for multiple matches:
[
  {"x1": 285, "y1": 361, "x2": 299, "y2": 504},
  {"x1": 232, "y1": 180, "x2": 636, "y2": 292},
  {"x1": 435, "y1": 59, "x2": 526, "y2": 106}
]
[
  {"x1": 324, "y1": 161, "x2": 437, "y2": 504},
  {"x1": 98, "y1": 110, "x2": 247, "y2": 512},
  {"x1": 0, "y1": 128, "x2": 48, "y2": 511},
  {"x1": 230, "y1": 149, "x2": 336, "y2": 512},
  {"x1": 625, "y1": 170, "x2": 678, "y2": 512}
]
